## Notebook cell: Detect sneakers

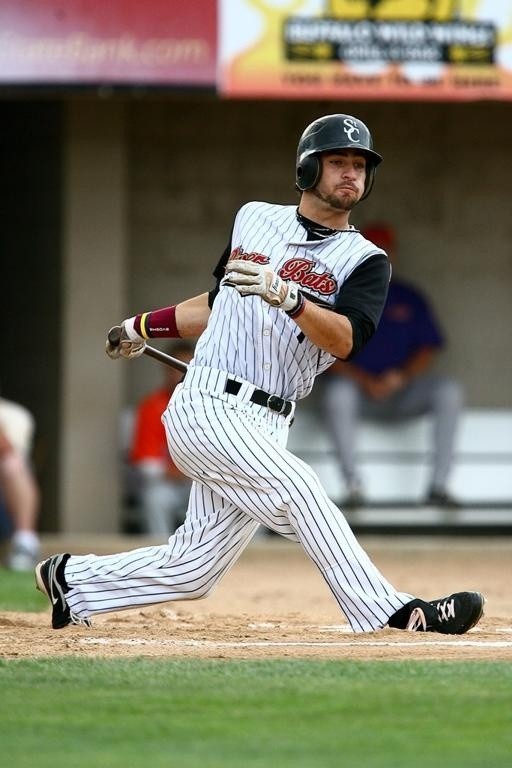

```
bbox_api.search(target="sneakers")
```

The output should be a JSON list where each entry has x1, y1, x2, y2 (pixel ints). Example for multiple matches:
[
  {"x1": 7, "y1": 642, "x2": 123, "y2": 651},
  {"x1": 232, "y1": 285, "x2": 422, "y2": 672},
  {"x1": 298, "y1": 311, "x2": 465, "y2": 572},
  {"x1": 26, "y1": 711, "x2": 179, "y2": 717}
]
[
  {"x1": 390, "y1": 591, "x2": 484, "y2": 633},
  {"x1": 35, "y1": 553, "x2": 92, "y2": 629}
]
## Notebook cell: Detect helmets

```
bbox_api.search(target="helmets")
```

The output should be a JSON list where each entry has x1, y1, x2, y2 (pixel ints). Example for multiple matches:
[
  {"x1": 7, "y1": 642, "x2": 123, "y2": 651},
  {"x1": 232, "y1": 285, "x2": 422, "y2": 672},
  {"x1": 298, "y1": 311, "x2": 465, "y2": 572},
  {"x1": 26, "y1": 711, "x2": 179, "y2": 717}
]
[{"x1": 295, "y1": 113, "x2": 381, "y2": 201}]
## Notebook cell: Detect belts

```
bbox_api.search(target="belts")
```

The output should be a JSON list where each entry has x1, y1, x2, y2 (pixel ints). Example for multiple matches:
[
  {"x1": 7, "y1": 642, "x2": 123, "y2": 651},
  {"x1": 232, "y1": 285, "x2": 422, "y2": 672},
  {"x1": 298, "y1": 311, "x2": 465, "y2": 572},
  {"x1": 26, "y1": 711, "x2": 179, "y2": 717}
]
[{"x1": 226, "y1": 374, "x2": 295, "y2": 425}]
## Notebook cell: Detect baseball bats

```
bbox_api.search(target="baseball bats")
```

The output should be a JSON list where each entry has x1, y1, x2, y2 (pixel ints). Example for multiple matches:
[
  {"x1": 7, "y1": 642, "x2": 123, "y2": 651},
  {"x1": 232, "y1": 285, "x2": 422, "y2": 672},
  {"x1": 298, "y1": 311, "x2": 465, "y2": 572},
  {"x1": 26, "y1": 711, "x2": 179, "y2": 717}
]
[{"x1": 107, "y1": 326, "x2": 187, "y2": 372}]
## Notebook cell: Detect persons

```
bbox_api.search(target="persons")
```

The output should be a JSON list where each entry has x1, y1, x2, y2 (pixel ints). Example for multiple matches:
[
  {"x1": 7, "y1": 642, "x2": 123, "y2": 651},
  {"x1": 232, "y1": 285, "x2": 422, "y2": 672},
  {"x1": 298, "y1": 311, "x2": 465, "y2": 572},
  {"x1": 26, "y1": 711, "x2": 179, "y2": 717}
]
[
  {"x1": 318, "y1": 219, "x2": 468, "y2": 510},
  {"x1": 0, "y1": 400, "x2": 45, "y2": 576},
  {"x1": 128, "y1": 342, "x2": 201, "y2": 534},
  {"x1": 34, "y1": 111, "x2": 486, "y2": 633}
]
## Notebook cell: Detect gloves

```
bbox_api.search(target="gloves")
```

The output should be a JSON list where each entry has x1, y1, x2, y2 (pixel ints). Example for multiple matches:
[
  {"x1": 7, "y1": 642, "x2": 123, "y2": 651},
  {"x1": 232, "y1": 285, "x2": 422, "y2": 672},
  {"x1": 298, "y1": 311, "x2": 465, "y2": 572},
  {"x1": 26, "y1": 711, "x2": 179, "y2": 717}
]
[
  {"x1": 105, "y1": 317, "x2": 146, "y2": 359},
  {"x1": 224, "y1": 260, "x2": 300, "y2": 314}
]
